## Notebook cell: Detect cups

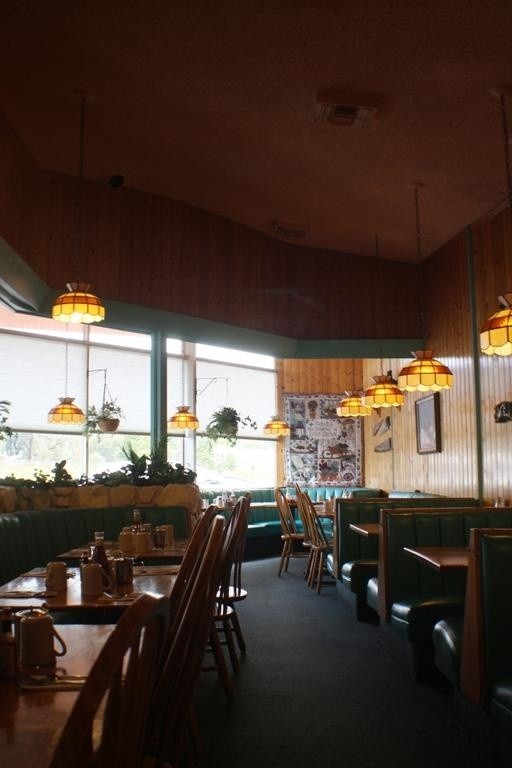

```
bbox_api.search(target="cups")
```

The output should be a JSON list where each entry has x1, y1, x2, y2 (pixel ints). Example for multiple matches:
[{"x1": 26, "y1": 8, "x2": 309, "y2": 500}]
[
  {"x1": 45, "y1": 562, "x2": 67, "y2": 591},
  {"x1": 119, "y1": 525, "x2": 175, "y2": 552},
  {"x1": 80, "y1": 563, "x2": 113, "y2": 597},
  {"x1": 14, "y1": 606, "x2": 68, "y2": 671},
  {"x1": 114, "y1": 558, "x2": 134, "y2": 586}
]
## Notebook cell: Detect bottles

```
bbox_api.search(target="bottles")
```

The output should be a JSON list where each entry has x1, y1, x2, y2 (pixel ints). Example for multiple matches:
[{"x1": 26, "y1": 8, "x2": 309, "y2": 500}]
[
  {"x1": 323, "y1": 487, "x2": 353, "y2": 514},
  {"x1": 79, "y1": 551, "x2": 87, "y2": 579},
  {"x1": 91, "y1": 529, "x2": 112, "y2": 588},
  {"x1": 130, "y1": 508, "x2": 141, "y2": 532},
  {"x1": 495, "y1": 496, "x2": 505, "y2": 508},
  {"x1": 216, "y1": 494, "x2": 236, "y2": 507}
]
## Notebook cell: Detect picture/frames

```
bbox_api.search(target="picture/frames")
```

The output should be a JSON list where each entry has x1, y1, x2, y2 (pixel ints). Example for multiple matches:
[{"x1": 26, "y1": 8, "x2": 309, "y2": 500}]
[{"x1": 412, "y1": 390, "x2": 443, "y2": 456}]
